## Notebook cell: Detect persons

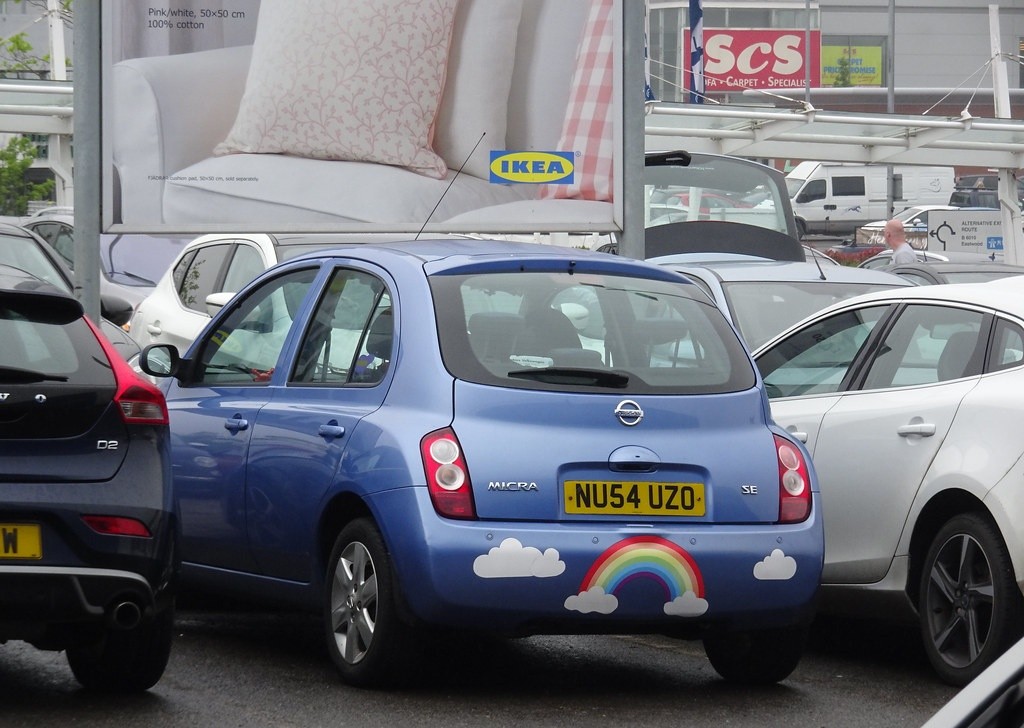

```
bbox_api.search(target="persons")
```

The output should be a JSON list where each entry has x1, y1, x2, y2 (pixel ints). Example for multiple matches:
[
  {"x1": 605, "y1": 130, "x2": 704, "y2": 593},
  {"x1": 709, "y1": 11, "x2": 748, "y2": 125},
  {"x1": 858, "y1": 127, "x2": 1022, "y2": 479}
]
[{"x1": 884, "y1": 220, "x2": 920, "y2": 284}]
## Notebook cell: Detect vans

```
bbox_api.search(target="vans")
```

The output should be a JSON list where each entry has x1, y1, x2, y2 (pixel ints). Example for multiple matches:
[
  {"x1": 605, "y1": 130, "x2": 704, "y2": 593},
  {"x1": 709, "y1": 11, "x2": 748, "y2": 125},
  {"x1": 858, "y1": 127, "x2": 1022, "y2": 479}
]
[{"x1": 754, "y1": 159, "x2": 954, "y2": 239}]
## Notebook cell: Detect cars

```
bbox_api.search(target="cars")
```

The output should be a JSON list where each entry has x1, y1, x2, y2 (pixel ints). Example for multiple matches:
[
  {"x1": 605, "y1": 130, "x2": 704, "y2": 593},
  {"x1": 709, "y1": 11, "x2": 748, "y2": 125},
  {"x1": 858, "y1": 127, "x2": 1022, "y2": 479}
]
[
  {"x1": 139, "y1": 239, "x2": 825, "y2": 681},
  {"x1": 860, "y1": 202, "x2": 955, "y2": 242},
  {"x1": 116, "y1": 225, "x2": 387, "y2": 374},
  {"x1": 750, "y1": 284, "x2": 1024, "y2": 681},
  {"x1": 0, "y1": 220, "x2": 176, "y2": 694}
]
